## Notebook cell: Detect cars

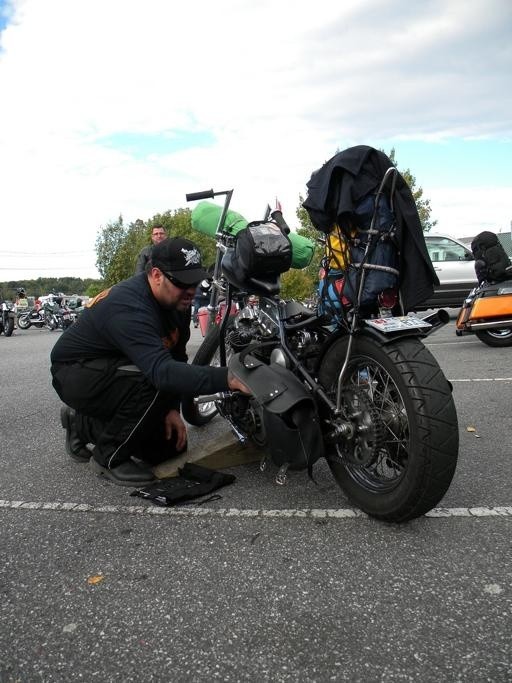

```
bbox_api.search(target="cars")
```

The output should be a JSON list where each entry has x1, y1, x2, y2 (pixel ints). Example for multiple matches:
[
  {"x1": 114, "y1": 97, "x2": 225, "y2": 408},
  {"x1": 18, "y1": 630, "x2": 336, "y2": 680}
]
[{"x1": 410, "y1": 234, "x2": 512, "y2": 312}]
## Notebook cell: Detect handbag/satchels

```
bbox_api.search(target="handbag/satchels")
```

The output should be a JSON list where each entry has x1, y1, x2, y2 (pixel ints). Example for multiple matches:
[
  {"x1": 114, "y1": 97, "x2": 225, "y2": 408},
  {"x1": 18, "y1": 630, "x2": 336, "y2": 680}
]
[{"x1": 219, "y1": 350, "x2": 329, "y2": 487}]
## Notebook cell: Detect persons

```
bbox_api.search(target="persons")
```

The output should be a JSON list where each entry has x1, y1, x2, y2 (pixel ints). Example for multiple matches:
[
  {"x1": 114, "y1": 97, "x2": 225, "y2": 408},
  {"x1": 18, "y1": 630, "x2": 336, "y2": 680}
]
[
  {"x1": 192, "y1": 283, "x2": 209, "y2": 326},
  {"x1": 133, "y1": 223, "x2": 169, "y2": 274},
  {"x1": 50, "y1": 236, "x2": 251, "y2": 484}
]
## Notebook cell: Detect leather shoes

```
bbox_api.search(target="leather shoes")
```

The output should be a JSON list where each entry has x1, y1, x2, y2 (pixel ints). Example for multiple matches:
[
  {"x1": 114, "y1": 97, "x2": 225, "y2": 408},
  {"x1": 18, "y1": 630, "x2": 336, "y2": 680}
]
[
  {"x1": 60, "y1": 405, "x2": 92, "y2": 462},
  {"x1": 89, "y1": 452, "x2": 158, "y2": 485}
]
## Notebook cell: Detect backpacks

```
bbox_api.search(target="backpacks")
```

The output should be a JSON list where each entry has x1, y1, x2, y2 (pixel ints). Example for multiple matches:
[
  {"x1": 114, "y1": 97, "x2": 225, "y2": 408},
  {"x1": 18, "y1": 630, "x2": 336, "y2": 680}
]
[
  {"x1": 312, "y1": 185, "x2": 401, "y2": 321},
  {"x1": 469, "y1": 229, "x2": 512, "y2": 285}
]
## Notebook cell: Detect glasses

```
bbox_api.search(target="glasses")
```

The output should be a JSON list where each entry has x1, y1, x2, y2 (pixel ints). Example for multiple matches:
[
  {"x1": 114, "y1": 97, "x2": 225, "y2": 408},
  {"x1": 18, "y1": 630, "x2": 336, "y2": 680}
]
[{"x1": 159, "y1": 267, "x2": 202, "y2": 290}]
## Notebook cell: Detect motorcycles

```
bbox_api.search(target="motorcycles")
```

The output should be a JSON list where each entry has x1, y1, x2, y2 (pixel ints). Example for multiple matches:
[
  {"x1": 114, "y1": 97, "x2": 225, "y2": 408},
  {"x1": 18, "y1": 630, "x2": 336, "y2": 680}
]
[
  {"x1": 0, "y1": 298, "x2": 92, "y2": 336},
  {"x1": 456, "y1": 260, "x2": 512, "y2": 348},
  {"x1": 182, "y1": 166, "x2": 459, "y2": 525}
]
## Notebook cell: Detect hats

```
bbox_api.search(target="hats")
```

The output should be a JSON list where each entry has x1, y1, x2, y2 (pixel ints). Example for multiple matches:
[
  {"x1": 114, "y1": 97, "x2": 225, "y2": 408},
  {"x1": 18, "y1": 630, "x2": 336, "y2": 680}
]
[{"x1": 151, "y1": 237, "x2": 213, "y2": 283}]
